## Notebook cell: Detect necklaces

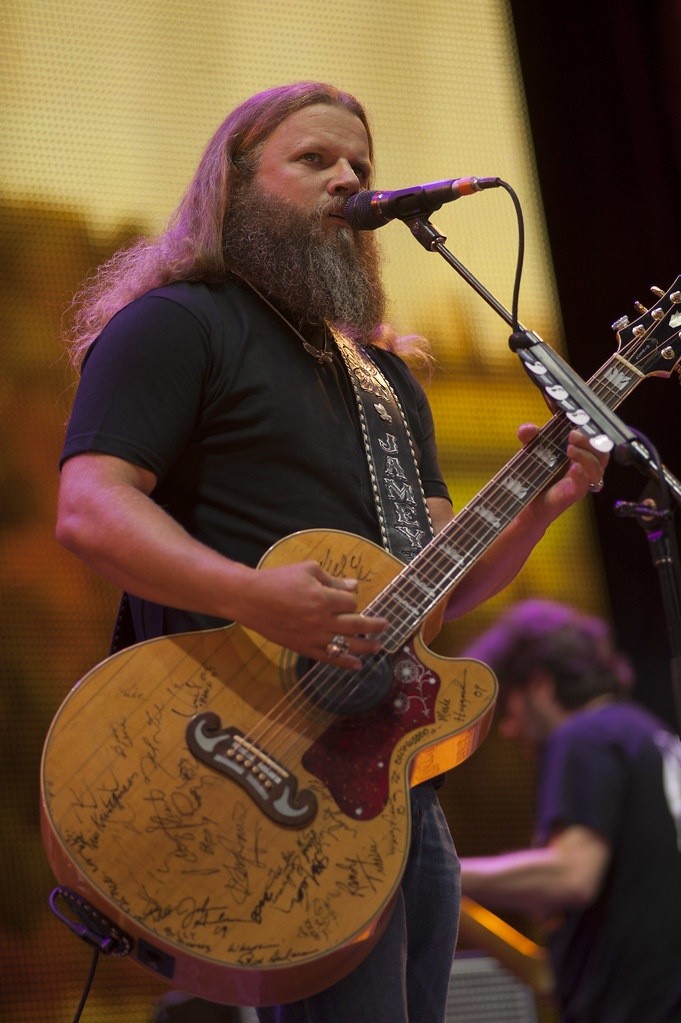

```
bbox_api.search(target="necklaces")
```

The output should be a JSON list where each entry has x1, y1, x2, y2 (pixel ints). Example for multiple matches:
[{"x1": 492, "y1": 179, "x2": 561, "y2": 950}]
[{"x1": 234, "y1": 269, "x2": 335, "y2": 365}]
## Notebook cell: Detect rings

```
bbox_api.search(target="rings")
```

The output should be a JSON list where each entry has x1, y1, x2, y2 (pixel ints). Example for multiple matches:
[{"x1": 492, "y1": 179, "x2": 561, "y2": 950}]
[
  {"x1": 325, "y1": 634, "x2": 349, "y2": 656},
  {"x1": 589, "y1": 477, "x2": 604, "y2": 493}
]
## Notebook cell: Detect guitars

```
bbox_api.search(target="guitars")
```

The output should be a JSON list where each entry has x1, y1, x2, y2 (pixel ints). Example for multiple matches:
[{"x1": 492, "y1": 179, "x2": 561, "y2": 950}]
[{"x1": 37, "y1": 273, "x2": 681, "y2": 1009}]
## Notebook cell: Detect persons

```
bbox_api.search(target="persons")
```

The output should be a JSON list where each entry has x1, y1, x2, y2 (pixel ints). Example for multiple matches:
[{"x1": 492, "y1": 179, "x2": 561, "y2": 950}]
[
  {"x1": 459, "y1": 599, "x2": 681, "y2": 1023},
  {"x1": 55, "y1": 79, "x2": 611, "y2": 1023}
]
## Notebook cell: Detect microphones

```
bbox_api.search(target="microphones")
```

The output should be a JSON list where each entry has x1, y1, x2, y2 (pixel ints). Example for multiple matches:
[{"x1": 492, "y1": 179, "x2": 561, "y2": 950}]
[{"x1": 344, "y1": 177, "x2": 502, "y2": 232}]
